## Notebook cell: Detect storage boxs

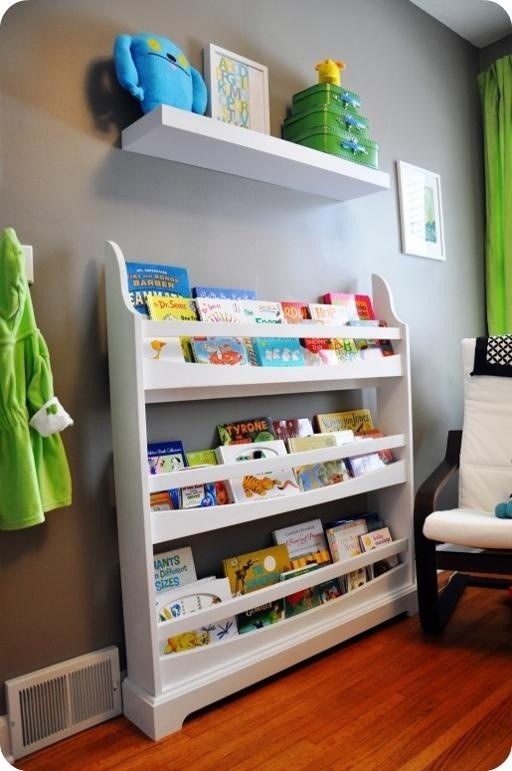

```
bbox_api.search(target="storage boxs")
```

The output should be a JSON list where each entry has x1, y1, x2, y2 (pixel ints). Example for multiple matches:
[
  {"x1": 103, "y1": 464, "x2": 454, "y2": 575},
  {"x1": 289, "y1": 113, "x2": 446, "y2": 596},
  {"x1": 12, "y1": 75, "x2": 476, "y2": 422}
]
[{"x1": 283, "y1": 81, "x2": 381, "y2": 171}]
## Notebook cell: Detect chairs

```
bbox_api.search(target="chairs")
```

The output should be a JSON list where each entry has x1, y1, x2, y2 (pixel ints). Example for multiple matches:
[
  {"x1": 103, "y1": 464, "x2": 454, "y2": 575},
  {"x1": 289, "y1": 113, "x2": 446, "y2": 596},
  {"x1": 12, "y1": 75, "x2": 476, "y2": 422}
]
[{"x1": 411, "y1": 334, "x2": 512, "y2": 639}]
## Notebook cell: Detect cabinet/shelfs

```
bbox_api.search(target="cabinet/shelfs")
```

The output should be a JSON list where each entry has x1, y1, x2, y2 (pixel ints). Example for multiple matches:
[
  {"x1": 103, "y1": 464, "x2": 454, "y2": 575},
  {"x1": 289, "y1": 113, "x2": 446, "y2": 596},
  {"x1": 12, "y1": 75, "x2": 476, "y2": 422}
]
[{"x1": 103, "y1": 241, "x2": 423, "y2": 745}]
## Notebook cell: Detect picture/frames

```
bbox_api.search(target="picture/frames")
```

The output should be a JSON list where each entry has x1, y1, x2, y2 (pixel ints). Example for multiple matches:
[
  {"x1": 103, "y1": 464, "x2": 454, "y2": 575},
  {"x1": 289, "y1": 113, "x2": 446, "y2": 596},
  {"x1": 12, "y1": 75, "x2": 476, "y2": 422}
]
[
  {"x1": 396, "y1": 158, "x2": 448, "y2": 261},
  {"x1": 203, "y1": 41, "x2": 273, "y2": 139}
]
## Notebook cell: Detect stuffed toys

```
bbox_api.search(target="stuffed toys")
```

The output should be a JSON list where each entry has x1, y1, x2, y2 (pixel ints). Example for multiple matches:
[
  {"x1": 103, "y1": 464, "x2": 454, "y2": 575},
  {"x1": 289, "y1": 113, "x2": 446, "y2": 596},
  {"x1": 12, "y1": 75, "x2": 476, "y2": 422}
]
[
  {"x1": 115, "y1": 31, "x2": 208, "y2": 114},
  {"x1": 495, "y1": 499, "x2": 512, "y2": 519},
  {"x1": 316, "y1": 59, "x2": 345, "y2": 87}
]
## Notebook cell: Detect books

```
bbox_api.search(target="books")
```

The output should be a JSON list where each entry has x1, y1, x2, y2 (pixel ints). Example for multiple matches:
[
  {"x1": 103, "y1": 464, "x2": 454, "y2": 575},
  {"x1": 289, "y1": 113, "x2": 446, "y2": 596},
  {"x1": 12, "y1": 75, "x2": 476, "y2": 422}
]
[{"x1": 128, "y1": 263, "x2": 402, "y2": 655}]
